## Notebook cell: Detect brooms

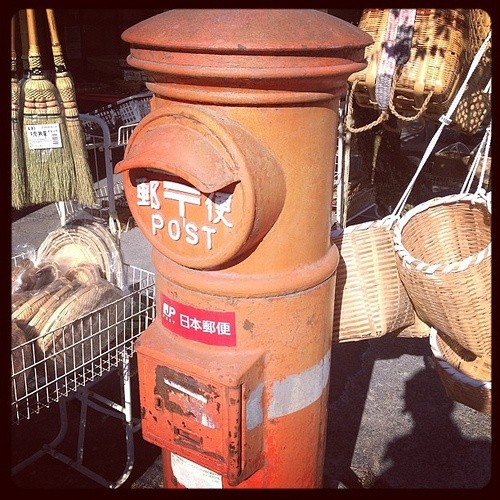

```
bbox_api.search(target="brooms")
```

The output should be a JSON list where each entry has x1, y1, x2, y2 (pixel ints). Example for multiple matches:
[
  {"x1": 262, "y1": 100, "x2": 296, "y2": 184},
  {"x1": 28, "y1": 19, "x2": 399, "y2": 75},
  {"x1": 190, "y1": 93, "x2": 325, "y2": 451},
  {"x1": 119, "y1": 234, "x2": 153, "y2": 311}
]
[{"x1": 10, "y1": 6, "x2": 99, "y2": 211}]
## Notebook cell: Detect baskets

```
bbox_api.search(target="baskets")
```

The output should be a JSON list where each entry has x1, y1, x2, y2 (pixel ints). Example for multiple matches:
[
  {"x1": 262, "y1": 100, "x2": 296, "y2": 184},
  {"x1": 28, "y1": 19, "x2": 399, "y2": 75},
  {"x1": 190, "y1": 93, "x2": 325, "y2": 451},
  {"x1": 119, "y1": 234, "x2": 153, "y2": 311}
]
[
  {"x1": 388, "y1": 310, "x2": 430, "y2": 337},
  {"x1": 418, "y1": 10, "x2": 491, "y2": 136},
  {"x1": 330, "y1": 215, "x2": 414, "y2": 343},
  {"x1": 394, "y1": 193, "x2": 491, "y2": 357},
  {"x1": 429, "y1": 327, "x2": 492, "y2": 417},
  {"x1": 12, "y1": 249, "x2": 156, "y2": 426},
  {"x1": 346, "y1": 9, "x2": 468, "y2": 134},
  {"x1": 82, "y1": 92, "x2": 151, "y2": 136}
]
